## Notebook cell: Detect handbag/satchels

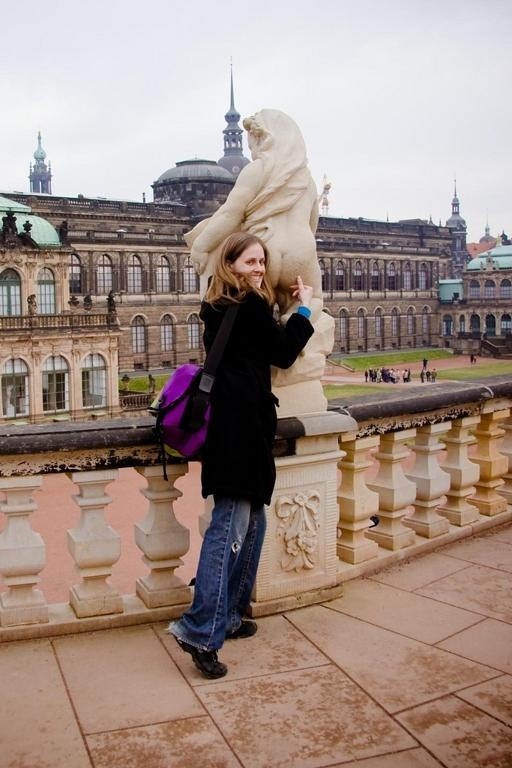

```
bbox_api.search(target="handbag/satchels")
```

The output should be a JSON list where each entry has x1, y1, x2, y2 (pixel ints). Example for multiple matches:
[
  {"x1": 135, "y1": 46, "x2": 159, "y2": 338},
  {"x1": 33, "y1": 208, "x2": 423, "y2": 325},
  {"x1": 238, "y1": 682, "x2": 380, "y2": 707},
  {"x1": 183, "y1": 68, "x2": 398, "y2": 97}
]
[{"x1": 147, "y1": 363, "x2": 214, "y2": 462}]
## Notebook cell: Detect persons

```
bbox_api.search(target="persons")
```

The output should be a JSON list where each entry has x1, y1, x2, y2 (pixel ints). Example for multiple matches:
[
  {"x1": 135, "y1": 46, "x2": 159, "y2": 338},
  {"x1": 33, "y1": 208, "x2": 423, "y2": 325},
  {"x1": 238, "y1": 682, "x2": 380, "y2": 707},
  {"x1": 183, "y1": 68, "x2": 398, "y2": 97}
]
[
  {"x1": 105, "y1": 289, "x2": 116, "y2": 323},
  {"x1": 426, "y1": 368, "x2": 431, "y2": 382},
  {"x1": 431, "y1": 367, "x2": 438, "y2": 382},
  {"x1": 161, "y1": 229, "x2": 318, "y2": 680},
  {"x1": 29, "y1": 293, "x2": 38, "y2": 317},
  {"x1": 422, "y1": 357, "x2": 428, "y2": 370},
  {"x1": 59, "y1": 217, "x2": 69, "y2": 242},
  {"x1": 421, "y1": 369, "x2": 426, "y2": 383},
  {"x1": 363, "y1": 365, "x2": 412, "y2": 383},
  {"x1": 186, "y1": 105, "x2": 327, "y2": 360}
]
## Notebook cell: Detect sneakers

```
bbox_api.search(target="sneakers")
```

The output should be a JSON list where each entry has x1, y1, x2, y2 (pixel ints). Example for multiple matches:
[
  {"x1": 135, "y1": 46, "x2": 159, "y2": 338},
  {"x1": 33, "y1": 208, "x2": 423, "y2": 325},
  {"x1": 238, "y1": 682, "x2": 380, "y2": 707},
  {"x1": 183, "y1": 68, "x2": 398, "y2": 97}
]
[
  {"x1": 224, "y1": 621, "x2": 259, "y2": 641},
  {"x1": 174, "y1": 632, "x2": 227, "y2": 679}
]
